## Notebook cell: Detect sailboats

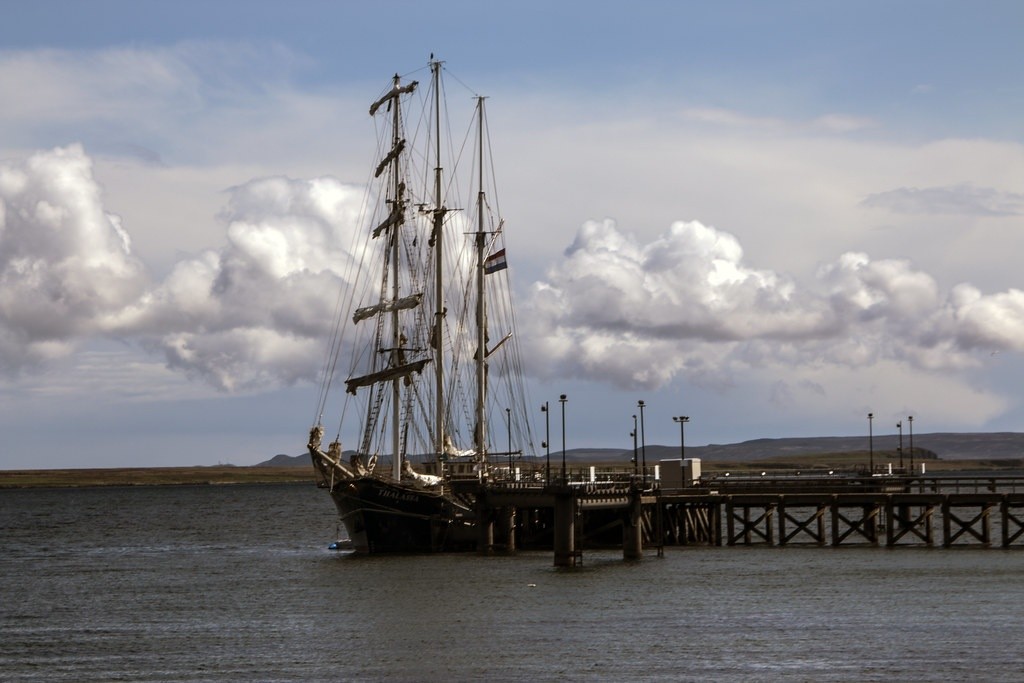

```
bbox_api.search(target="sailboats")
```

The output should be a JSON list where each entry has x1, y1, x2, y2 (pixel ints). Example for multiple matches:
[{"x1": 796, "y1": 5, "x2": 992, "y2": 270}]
[{"x1": 307, "y1": 55, "x2": 708, "y2": 556}]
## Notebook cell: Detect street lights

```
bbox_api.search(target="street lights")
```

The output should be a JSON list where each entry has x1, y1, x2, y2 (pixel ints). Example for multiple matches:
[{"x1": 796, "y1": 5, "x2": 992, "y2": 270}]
[
  {"x1": 541, "y1": 401, "x2": 550, "y2": 486},
  {"x1": 559, "y1": 394, "x2": 568, "y2": 485},
  {"x1": 673, "y1": 416, "x2": 689, "y2": 495},
  {"x1": 868, "y1": 414, "x2": 874, "y2": 477},
  {"x1": 909, "y1": 416, "x2": 914, "y2": 477},
  {"x1": 630, "y1": 415, "x2": 638, "y2": 475},
  {"x1": 506, "y1": 408, "x2": 510, "y2": 481},
  {"x1": 897, "y1": 421, "x2": 903, "y2": 467},
  {"x1": 638, "y1": 400, "x2": 647, "y2": 489}
]
